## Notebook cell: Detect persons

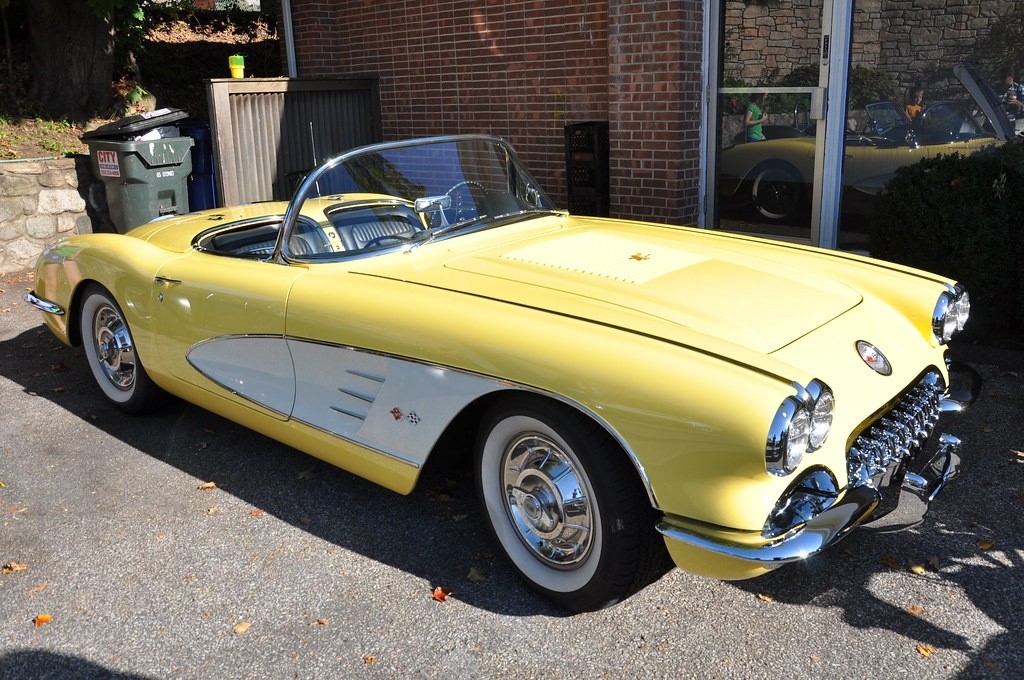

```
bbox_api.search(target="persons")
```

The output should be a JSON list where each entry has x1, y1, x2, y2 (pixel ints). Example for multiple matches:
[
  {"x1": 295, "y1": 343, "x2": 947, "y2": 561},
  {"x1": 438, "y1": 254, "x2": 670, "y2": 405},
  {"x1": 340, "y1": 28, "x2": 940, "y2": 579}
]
[
  {"x1": 743, "y1": 83, "x2": 770, "y2": 142},
  {"x1": 991, "y1": 65, "x2": 1024, "y2": 137},
  {"x1": 905, "y1": 86, "x2": 924, "y2": 120}
]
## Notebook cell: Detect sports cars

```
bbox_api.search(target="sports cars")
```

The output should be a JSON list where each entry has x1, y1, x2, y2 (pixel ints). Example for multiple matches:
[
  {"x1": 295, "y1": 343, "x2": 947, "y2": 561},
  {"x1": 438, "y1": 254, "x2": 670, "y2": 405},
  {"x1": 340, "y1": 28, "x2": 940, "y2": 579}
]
[
  {"x1": 716, "y1": 59, "x2": 1018, "y2": 230},
  {"x1": 22, "y1": 131, "x2": 969, "y2": 616}
]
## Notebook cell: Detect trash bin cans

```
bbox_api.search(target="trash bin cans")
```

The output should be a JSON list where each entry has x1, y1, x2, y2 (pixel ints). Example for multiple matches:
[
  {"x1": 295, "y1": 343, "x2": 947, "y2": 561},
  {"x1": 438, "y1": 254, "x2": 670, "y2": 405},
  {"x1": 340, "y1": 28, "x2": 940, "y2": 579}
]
[
  {"x1": 181, "y1": 106, "x2": 217, "y2": 212},
  {"x1": 72, "y1": 105, "x2": 194, "y2": 235}
]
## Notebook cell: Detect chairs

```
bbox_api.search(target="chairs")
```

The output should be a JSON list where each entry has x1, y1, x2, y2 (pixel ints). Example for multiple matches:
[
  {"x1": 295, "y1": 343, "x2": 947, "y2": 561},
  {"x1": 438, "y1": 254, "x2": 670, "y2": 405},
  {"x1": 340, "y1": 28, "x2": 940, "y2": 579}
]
[
  {"x1": 192, "y1": 212, "x2": 334, "y2": 259},
  {"x1": 324, "y1": 198, "x2": 417, "y2": 253}
]
